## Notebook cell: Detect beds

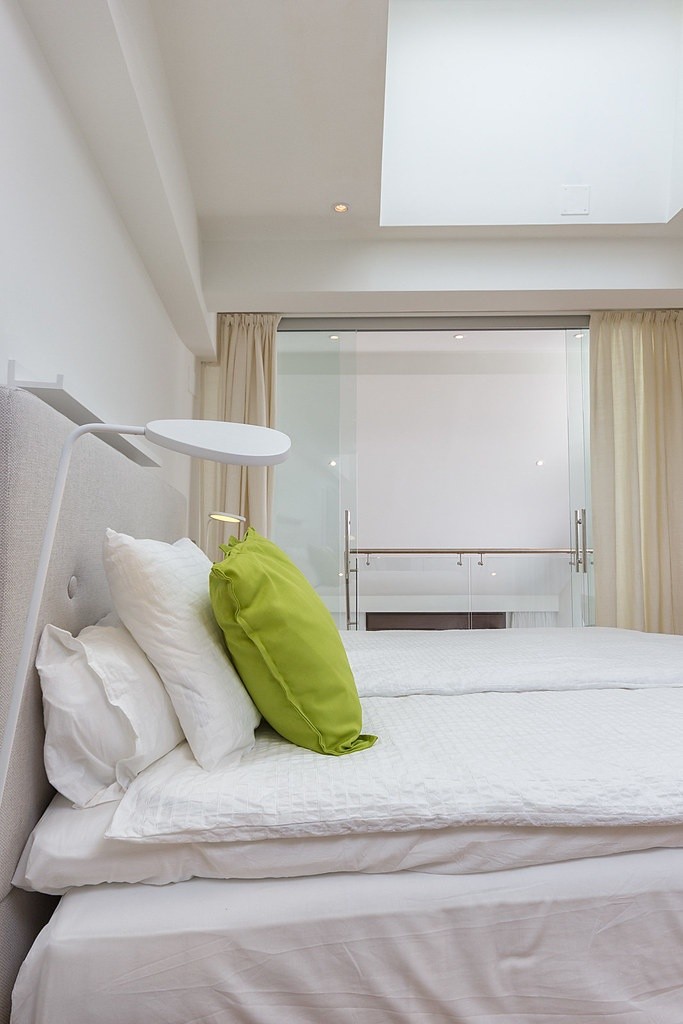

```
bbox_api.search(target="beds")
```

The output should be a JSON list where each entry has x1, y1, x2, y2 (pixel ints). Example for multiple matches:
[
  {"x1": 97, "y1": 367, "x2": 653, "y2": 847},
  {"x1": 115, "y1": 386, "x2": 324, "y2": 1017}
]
[{"x1": 0, "y1": 380, "x2": 683, "y2": 1023}]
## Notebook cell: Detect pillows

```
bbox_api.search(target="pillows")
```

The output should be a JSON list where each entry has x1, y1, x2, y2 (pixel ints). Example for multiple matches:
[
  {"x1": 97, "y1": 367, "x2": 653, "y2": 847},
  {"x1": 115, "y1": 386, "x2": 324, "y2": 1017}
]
[
  {"x1": 36, "y1": 616, "x2": 185, "y2": 806},
  {"x1": 101, "y1": 528, "x2": 262, "y2": 770},
  {"x1": 209, "y1": 530, "x2": 379, "y2": 758}
]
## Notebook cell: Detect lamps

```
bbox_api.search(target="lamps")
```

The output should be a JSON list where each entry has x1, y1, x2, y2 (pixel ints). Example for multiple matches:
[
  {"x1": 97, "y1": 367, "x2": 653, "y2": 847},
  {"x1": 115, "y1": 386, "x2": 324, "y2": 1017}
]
[{"x1": 0, "y1": 414, "x2": 295, "y2": 811}]
